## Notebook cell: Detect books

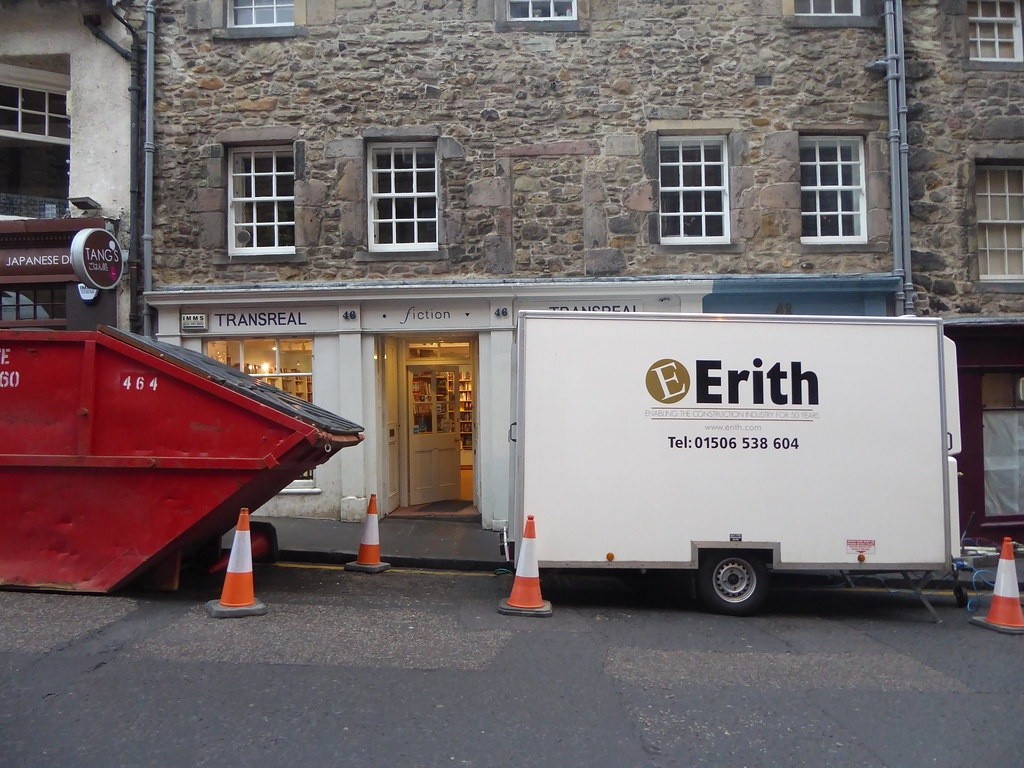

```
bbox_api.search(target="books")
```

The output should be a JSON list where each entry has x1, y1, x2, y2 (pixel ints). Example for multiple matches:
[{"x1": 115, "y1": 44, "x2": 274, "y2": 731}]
[{"x1": 228, "y1": 357, "x2": 476, "y2": 485}]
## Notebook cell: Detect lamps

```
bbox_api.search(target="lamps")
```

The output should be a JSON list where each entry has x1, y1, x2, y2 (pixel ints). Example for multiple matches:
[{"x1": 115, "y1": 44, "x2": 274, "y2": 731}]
[{"x1": 67, "y1": 197, "x2": 102, "y2": 210}]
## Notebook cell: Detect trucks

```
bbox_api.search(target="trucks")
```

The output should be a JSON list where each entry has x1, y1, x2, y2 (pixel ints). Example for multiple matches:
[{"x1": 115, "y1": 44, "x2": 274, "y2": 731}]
[{"x1": 500, "y1": 308, "x2": 1023, "y2": 617}]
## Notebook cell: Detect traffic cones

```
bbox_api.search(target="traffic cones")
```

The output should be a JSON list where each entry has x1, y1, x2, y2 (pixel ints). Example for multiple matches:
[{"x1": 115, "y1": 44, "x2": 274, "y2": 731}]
[
  {"x1": 340, "y1": 493, "x2": 390, "y2": 574},
  {"x1": 203, "y1": 504, "x2": 268, "y2": 618},
  {"x1": 970, "y1": 533, "x2": 1023, "y2": 635},
  {"x1": 496, "y1": 515, "x2": 554, "y2": 616}
]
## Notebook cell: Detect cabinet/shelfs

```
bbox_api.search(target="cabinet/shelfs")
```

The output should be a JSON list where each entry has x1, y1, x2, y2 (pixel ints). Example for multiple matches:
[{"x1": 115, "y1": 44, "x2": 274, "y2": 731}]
[
  {"x1": 412, "y1": 373, "x2": 473, "y2": 450},
  {"x1": 243, "y1": 367, "x2": 314, "y2": 404}
]
